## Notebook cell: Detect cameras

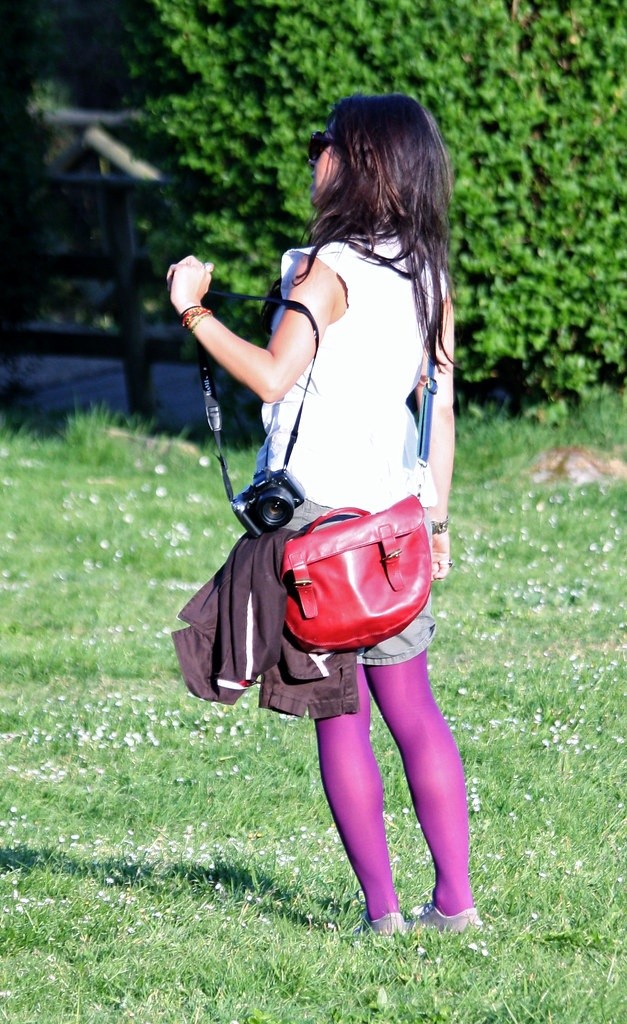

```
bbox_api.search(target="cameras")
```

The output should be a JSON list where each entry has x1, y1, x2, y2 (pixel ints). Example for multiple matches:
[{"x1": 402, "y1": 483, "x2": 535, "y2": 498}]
[{"x1": 230, "y1": 468, "x2": 305, "y2": 538}]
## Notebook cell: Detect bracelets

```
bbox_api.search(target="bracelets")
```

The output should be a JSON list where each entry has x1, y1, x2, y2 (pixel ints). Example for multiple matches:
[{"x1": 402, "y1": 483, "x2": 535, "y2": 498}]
[
  {"x1": 181, "y1": 306, "x2": 212, "y2": 339},
  {"x1": 431, "y1": 515, "x2": 448, "y2": 534}
]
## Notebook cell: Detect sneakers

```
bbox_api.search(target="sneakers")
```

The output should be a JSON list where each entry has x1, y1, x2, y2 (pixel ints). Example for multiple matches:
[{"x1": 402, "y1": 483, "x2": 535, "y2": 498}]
[
  {"x1": 404, "y1": 900, "x2": 484, "y2": 936},
  {"x1": 351, "y1": 888, "x2": 406, "y2": 941}
]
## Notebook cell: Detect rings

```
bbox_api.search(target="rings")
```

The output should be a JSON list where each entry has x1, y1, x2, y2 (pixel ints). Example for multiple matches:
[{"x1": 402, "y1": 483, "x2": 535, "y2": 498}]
[{"x1": 439, "y1": 559, "x2": 453, "y2": 568}]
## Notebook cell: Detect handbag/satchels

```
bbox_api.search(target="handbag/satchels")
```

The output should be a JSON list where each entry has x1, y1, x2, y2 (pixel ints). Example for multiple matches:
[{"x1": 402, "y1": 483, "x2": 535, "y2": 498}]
[{"x1": 279, "y1": 495, "x2": 432, "y2": 652}]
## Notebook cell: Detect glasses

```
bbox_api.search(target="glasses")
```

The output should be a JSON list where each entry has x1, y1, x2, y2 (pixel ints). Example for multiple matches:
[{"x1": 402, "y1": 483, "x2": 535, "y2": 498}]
[{"x1": 309, "y1": 132, "x2": 338, "y2": 160}]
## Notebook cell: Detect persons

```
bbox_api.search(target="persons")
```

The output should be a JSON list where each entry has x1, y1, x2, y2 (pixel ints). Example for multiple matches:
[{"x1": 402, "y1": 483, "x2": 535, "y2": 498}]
[{"x1": 167, "y1": 96, "x2": 483, "y2": 937}]
